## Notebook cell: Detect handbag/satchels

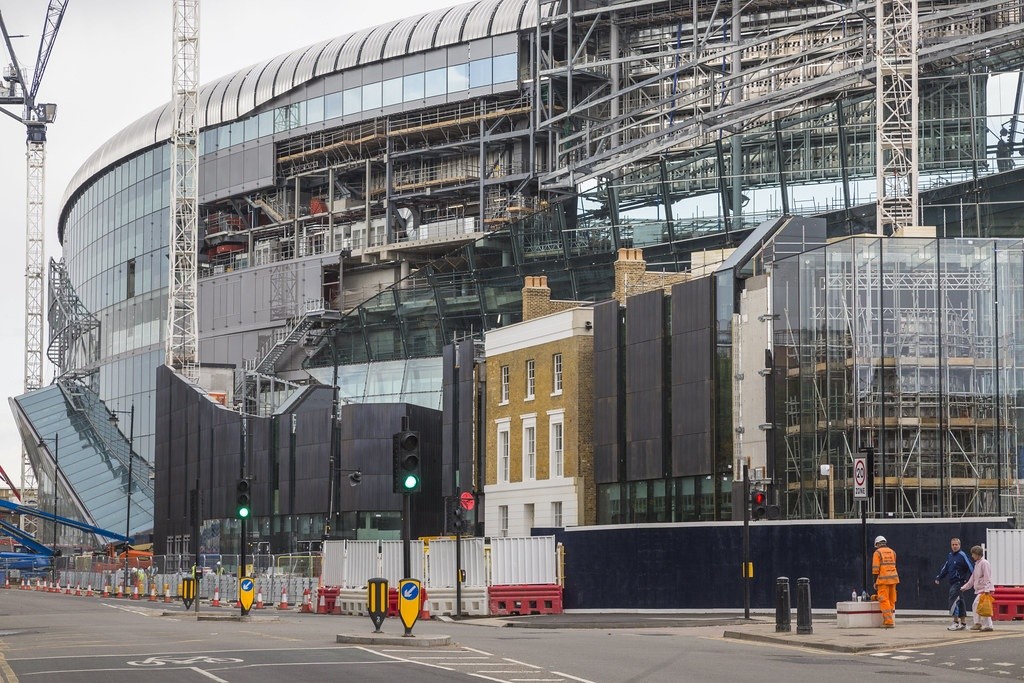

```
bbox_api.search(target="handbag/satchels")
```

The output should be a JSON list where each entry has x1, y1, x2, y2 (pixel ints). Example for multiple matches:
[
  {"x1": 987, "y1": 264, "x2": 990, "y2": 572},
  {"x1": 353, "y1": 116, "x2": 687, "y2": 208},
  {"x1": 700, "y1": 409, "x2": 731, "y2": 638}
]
[{"x1": 976, "y1": 592, "x2": 995, "y2": 617}]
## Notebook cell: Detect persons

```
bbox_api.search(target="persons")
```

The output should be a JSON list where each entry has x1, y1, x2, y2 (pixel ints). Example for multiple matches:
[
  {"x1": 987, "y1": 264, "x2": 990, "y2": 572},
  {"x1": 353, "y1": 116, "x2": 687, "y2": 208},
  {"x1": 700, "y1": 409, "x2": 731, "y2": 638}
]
[
  {"x1": 935, "y1": 538, "x2": 974, "y2": 631},
  {"x1": 131, "y1": 561, "x2": 232, "y2": 596},
  {"x1": 872, "y1": 536, "x2": 899, "y2": 628},
  {"x1": 960, "y1": 545, "x2": 995, "y2": 632}
]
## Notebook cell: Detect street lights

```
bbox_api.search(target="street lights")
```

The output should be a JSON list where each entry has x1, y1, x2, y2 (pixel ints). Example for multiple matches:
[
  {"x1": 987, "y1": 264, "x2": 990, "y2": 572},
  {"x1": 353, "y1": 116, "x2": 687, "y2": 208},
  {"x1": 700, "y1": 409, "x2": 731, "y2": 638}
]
[
  {"x1": 106, "y1": 410, "x2": 135, "y2": 592},
  {"x1": 36, "y1": 437, "x2": 58, "y2": 583},
  {"x1": 303, "y1": 333, "x2": 338, "y2": 539}
]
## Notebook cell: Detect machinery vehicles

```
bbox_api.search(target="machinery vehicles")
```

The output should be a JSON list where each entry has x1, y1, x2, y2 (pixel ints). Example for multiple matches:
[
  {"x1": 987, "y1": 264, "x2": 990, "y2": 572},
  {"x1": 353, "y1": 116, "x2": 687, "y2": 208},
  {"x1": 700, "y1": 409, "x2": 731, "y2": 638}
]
[{"x1": 0, "y1": 465, "x2": 152, "y2": 582}]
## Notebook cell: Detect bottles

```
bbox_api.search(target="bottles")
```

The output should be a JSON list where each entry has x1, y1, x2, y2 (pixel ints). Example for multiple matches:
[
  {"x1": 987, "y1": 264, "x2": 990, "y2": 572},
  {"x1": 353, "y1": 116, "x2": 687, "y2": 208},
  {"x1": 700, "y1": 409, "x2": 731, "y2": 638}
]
[
  {"x1": 862, "y1": 589, "x2": 866, "y2": 601},
  {"x1": 851, "y1": 589, "x2": 857, "y2": 602}
]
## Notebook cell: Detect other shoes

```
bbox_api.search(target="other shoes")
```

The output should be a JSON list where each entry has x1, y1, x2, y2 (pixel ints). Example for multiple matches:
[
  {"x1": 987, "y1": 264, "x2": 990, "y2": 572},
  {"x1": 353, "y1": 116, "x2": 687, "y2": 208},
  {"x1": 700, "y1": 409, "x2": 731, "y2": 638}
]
[
  {"x1": 879, "y1": 623, "x2": 895, "y2": 628},
  {"x1": 947, "y1": 621, "x2": 967, "y2": 631},
  {"x1": 969, "y1": 624, "x2": 994, "y2": 632}
]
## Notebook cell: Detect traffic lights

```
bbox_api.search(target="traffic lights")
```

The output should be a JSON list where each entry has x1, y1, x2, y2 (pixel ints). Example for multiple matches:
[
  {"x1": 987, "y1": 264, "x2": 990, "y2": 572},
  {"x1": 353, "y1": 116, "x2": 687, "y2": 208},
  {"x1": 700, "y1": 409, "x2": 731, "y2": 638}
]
[
  {"x1": 236, "y1": 479, "x2": 250, "y2": 521},
  {"x1": 454, "y1": 507, "x2": 467, "y2": 530},
  {"x1": 398, "y1": 432, "x2": 421, "y2": 491},
  {"x1": 751, "y1": 491, "x2": 781, "y2": 520}
]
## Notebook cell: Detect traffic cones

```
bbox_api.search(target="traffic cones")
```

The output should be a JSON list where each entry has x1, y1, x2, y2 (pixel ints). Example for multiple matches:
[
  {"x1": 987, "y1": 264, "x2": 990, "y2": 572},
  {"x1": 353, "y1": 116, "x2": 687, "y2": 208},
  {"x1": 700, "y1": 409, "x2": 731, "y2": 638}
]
[
  {"x1": 275, "y1": 586, "x2": 292, "y2": 610},
  {"x1": 63, "y1": 579, "x2": 72, "y2": 595},
  {"x1": 209, "y1": 586, "x2": 223, "y2": 607},
  {"x1": 130, "y1": 584, "x2": 141, "y2": 600},
  {"x1": 34, "y1": 578, "x2": 61, "y2": 593},
  {"x1": 3, "y1": 578, "x2": 12, "y2": 588},
  {"x1": 19, "y1": 577, "x2": 32, "y2": 590},
  {"x1": 147, "y1": 584, "x2": 172, "y2": 603},
  {"x1": 298, "y1": 589, "x2": 341, "y2": 615},
  {"x1": 101, "y1": 583, "x2": 111, "y2": 598},
  {"x1": 234, "y1": 592, "x2": 241, "y2": 607},
  {"x1": 419, "y1": 595, "x2": 432, "y2": 620},
  {"x1": 114, "y1": 582, "x2": 124, "y2": 598},
  {"x1": 85, "y1": 582, "x2": 94, "y2": 596},
  {"x1": 73, "y1": 583, "x2": 82, "y2": 597},
  {"x1": 253, "y1": 589, "x2": 265, "y2": 609}
]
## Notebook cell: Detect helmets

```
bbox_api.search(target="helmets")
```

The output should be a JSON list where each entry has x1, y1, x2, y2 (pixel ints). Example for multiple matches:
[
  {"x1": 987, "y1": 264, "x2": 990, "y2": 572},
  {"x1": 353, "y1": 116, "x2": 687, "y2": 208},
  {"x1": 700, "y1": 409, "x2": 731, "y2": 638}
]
[{"x1": 874, "y1": 535, "x2": 888, "y2": 548}]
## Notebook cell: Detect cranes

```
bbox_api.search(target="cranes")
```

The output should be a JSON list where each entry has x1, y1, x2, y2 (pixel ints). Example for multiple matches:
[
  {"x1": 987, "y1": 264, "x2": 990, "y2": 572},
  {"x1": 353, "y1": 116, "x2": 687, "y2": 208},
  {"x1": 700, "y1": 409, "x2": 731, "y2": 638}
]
[{"x1": 0, "y1": 0, "x2": 71, "y2": 538}]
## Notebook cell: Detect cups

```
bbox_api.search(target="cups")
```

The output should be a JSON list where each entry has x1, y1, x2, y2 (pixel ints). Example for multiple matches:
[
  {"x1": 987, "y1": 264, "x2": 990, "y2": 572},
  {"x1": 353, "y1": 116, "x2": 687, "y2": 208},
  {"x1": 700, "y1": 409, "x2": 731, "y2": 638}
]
[{"x1": 857, "y1": 596, "x2": 862, "y2": 602}]
